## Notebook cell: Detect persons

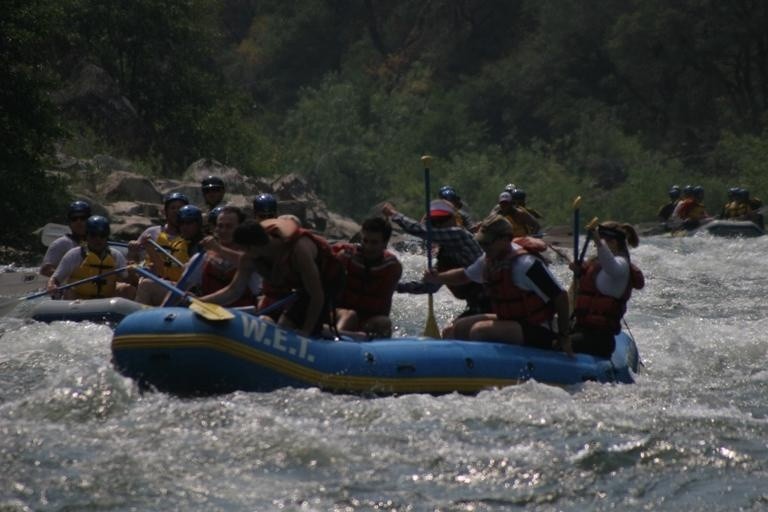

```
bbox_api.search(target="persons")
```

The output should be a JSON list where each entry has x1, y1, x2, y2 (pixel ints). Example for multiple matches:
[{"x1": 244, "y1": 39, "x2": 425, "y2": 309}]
[
  {"x1": 660, "y1": 184, "x2": 760, "y2": 223},
  {"x1": 420, "y1": 213, "x2": 575, "y2": 361},
  {"x1": 438, "y1": 180, "x2": 542, "y2": 240},
  {"x1": 564, "y1": 219, "x2": 638, "y2": 355},
  {"x1": 381, "y1": 201, "x2": 484, "y2": 338}
]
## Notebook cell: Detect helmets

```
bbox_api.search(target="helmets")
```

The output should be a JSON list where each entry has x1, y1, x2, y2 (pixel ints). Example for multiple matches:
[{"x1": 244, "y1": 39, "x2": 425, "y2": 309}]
[
  {"x1": 174, "y1": 204, "x2": 203, "y2": 225},
  {"x1": 67, "y1": 200, "x2": 92, "y2": 213},
  {"x1": 667, "y1": 184, "x2": 706, "y2": 195},
  {"x1": 253, "y1": 193, "x2": 278, "y2": 211},
  {"x1": 727, "y1": 188, "x2": 749, "y2": 200},
  {"x1": 165, "y1": 192, "x2": 190, "y2": 207},
  {"x1": 84, "y1": 215, "x2": 110, "y2": 232},
  {"x1": 201, "y1": 176, "x2": 226, "y2": 189}
]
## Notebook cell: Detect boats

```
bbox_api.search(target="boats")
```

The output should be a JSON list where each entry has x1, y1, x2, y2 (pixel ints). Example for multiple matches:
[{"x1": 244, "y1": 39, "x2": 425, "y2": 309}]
[
  {"x1": 24, "y1": 293, "x2": 155, "y2": 322},
  {"x1": 101, "y1": 300, "x2": 643, "y2": 397},
  {"x1": 641, "y1": 217, "x2": 714, "y2": 237},
  {"x1": 705, "y1": 217, "x2": 763, "y2": 237}
]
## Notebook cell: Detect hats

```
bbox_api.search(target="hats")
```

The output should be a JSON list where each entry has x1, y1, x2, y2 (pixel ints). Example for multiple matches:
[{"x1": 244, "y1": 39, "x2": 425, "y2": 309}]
[
  {"x1": 439, "y1": 186, "x2": 456, "y2": 197},
  {"x1": 423, "y1": 203, "x2": 457, "y2": 221},
  {"x1": 586, "y1": 221, "x2": 628, "y2": 240},
  {"x1": 472, "y1": 213, "x2": 514, "y2": 242},
  {"x1": 499, "y1": 182, "x2": 527, "y2": 203}
]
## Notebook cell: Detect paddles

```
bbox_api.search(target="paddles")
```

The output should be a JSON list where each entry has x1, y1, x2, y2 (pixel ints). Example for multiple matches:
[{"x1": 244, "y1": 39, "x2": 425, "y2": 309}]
[
  {"x1": 421, "y1": 156, "x2": 441, "y2": 339},
  {"x1": 41, "y1": 223, "x2": 171, "y2": 253},
  {"x1": 567, "y1": 216, "x2": 598, "y2": 316},
  {"x1": 131, "y1": 263, "x2": 235, "y2": 321},
  {"x1": 0, "y1": 265, "x2": 133, "y2": 317}
]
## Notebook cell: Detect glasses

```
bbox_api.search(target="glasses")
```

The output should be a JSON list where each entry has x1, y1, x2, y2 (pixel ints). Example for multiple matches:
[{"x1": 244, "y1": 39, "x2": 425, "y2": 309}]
[
  {"x1": 89, "y1": 232, "x2": 107, "y2": 239},
  {"x1": 71, "y1": 215, "x2": 87, "y2": 222},
  {"x1": 217, "y1": 222, "x2": 237, "y2": 229},
  {"x1": 362, "y1": 237, "x2": 381, "y2": 245}
]
